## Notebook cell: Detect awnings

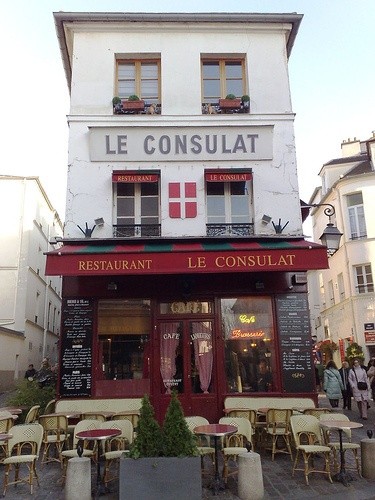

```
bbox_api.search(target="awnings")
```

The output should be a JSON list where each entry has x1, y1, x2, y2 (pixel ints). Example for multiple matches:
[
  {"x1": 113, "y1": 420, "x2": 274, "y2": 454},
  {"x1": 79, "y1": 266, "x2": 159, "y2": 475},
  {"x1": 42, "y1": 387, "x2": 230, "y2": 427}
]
[{"x1": 44, "y1": 238, "x2": 330, "y2": 275}]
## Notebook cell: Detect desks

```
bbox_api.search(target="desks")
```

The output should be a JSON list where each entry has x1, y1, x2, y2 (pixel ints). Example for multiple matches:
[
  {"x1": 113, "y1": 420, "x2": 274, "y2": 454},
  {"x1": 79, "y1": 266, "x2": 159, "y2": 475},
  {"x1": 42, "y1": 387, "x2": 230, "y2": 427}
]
[
  {"x1": 47, "y1": 411, "x2": 80, "y2": 460},
  {"x1": 85, "y1": 410, "x2": 114, "y2": 418},
  {"x1": 320, "y1": 420, "x2": 363, "y2": 488},
  {"x1": 258, "y1": 407, "x2": 279, "y2": 457},
  {"x1": 193, "y1": 423, "x2": 238, "y2": 496},
  {"x1": 2, "y1": 406, "x2": 29, "y2": 424},
  {"x1": 74, "y1": 429, "x2": 121, "y2": 500}
]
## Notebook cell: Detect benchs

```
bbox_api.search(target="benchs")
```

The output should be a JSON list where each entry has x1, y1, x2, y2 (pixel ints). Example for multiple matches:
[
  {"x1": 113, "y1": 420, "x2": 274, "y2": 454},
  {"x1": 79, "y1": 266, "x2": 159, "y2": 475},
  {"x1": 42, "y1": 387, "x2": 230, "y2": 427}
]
[
  {"x1": 52, "y1": 396, "x2": 150, "y2": 435},
  {"x1": 224, "y1": 397, "x2": 318, "y2": 409}
]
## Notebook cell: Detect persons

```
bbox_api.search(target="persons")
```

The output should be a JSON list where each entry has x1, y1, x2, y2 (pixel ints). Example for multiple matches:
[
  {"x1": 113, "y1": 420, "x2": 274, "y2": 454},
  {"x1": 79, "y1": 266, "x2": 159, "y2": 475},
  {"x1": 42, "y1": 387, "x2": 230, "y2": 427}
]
[
  {"x1": 24, "y1": 357, "x2": 54, "y2": 390},
  {"x1": 314, "y1": 357, "x2": 375, "y2": 420}
]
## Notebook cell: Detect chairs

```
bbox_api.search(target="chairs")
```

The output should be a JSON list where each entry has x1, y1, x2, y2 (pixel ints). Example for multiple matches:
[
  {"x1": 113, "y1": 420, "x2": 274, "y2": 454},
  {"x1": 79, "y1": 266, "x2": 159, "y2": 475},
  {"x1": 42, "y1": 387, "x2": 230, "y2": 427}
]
[
  {"x1": 184, "y1": 408, "x2": 361, "y2": 487},
  {"x1": 0, "y1": 405, "x2": 140, "y2": 497}
]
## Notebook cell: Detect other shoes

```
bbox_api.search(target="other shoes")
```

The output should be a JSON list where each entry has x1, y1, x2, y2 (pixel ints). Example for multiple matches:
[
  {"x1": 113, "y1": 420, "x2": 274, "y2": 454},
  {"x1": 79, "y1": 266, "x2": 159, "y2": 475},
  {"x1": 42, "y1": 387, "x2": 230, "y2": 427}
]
[{"x1": 363, "y1": 417, "x2": 368, "y2": 420}]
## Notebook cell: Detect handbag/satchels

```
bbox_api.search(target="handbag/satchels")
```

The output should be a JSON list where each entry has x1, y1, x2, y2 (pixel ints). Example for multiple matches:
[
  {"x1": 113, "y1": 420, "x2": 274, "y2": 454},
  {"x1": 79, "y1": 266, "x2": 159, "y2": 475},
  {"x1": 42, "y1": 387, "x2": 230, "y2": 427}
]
[{"x1": 357, "y1": 381, "x2": 367, "y2": 390}]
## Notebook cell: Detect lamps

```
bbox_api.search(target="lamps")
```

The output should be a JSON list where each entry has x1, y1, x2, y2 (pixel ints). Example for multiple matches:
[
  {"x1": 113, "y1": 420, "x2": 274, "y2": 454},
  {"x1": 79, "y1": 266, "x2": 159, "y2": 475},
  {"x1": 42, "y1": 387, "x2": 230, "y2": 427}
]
[
  {"x1": 48, "y1": 235, "x2": 62, "y2": 244},
  {"x1": 95, "y1": 217, "x2": 104, "y2": 226},
  {"x1": 301, "y1": 203, "x2": 343, "y2": 256},
  {"x1": 255, "y1": 281, "x2": 264, "y2": 288},
  {"x1": 106, "y1": 282, "x2": 117, "y2": 290},
  {"x1": 261, "y1": 215, "x2": 271, "y2": 224}
]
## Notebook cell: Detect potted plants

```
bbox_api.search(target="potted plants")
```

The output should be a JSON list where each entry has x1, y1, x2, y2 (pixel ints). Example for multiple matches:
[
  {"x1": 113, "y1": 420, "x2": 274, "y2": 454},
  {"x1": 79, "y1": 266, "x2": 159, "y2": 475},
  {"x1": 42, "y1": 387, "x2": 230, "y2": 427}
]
[
  {"x1": 122, "y1": 95, "x2": 145, "y2": 111},
  {"x1": 241, "y1": 95, "x2": 250, "y2": 109},
  {"x1": 119, "y1": 388, "x2": 204, "y2": 500},
  {"x1": 113, "y1": 96, "x2": 121, "y2": 111},
  {"x1": 219, "y1": 94, "x2": 240, "y2": 109}
]
